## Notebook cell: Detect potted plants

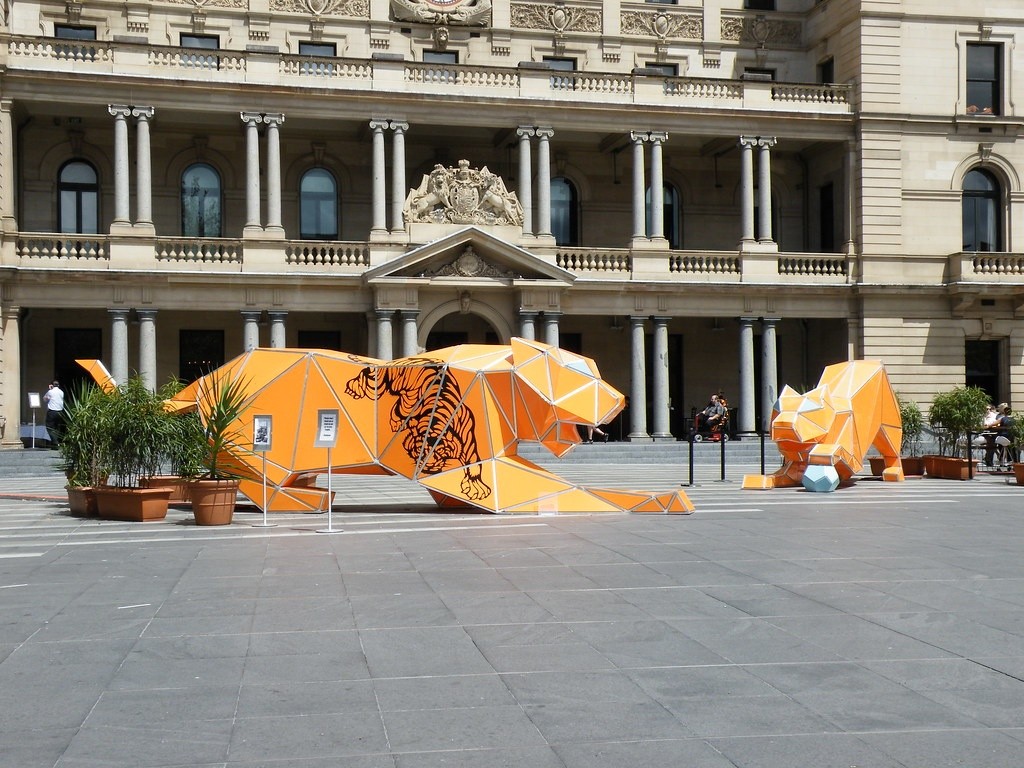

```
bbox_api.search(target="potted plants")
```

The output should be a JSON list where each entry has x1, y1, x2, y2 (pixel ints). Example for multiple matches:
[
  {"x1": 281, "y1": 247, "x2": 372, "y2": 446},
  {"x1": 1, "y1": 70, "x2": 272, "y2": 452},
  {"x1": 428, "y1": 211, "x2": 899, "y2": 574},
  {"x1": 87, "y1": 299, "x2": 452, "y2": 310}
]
[
  {"x1": 137, "y1": 412, "x2": 209, "y2": 500},
  {"x1": 921, "y1": 386, "x2": 991, "y2": 481},
  {"x1": 1007, "y1": 408, "x2": 1024, "y2": 486},
  {"x1": 867, "y1": 391, "x2": 925, "y2": 476},
  {"x1": 187, "y1": 370, "x2": 251, "y2": 527},
  {"x1": 62, "y1": 392, "x2": 109, "y2": 485},
  {"x1": 91, "y1": 370, "x2": 175, "y2": 523},
  {"x1": 58, "y1": 378, "x2": 95, "y2": 519}
]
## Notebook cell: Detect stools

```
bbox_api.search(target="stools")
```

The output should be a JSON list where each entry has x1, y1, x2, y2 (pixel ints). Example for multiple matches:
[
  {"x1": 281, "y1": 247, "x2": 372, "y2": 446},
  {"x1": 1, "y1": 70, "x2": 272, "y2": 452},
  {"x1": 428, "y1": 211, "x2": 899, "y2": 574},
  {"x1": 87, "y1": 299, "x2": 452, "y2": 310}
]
[
  {"x1": 957, "y1": 437, "x2": 968, "y2": 460},
  {"x1": 972, "y1": 437, "x2": 987, "y2": 471},
  {"x1": 995, "y1": 436, "x2": 1014, "y2": 465}
]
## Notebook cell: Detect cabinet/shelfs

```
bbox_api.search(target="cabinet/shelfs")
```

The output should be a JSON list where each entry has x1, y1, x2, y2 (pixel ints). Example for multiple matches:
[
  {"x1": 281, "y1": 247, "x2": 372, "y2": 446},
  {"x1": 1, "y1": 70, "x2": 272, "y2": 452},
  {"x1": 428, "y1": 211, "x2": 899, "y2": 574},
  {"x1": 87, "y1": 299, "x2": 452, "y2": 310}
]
[{"x1": 681, "y1": 417, "x2": 701, "y2": 440}]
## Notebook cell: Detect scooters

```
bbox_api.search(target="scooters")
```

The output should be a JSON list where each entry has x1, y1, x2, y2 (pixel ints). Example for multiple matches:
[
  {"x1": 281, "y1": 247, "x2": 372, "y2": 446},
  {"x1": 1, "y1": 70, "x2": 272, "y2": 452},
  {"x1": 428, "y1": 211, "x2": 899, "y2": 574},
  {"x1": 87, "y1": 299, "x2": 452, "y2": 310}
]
[{"x1": 689, "y1": 414, "x2": 728, "y2": 443}]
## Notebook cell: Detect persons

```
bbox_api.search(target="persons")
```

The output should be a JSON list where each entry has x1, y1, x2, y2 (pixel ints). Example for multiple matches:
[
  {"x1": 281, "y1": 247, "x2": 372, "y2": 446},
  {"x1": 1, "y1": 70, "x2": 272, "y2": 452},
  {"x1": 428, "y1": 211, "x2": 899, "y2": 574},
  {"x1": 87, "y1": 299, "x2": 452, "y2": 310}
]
[
  {"x1": 697, "y1": 394, "x2": 726, "y2": 440},
  {"x1": 980, "y1": 402, "x2": 1022, "y2": 472},
  {"x1": 586, "y1": 426, "x2": 609, "y2": 444},
  {"x1": 461, "y1": 290, "x2": 472, "y2": 312},
  {"x1": 43, "y1": 381, "x2": 64, "y2": 450}
]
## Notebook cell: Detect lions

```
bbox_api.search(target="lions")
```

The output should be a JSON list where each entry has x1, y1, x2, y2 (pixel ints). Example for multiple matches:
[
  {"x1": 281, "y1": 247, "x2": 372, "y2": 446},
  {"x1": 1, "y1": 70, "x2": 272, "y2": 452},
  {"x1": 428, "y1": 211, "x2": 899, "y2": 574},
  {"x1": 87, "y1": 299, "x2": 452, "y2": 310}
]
[{"x1": 412, "y1": 169, "x2": 453, "y2": 221}]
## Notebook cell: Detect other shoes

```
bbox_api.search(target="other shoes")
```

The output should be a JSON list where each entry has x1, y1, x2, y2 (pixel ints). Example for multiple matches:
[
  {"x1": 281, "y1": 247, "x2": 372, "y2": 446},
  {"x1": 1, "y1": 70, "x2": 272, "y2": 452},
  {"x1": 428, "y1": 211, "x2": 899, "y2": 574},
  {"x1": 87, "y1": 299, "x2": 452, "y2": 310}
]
[
  {"x1": 583, "y1": 439, "x2": 593, "y2": 444},
  {"x1": 1000, "y1": 464, "x2": 1006, "y2": 467},
  {"x1": 604, "y1": 434, "x2": 608, "y2": 443}
]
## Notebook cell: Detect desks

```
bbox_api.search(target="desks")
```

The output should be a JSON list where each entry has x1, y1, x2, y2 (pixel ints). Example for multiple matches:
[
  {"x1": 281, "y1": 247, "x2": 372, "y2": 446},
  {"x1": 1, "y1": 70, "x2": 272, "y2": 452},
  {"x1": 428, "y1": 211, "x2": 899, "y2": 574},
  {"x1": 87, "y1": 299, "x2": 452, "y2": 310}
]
[
  {"x1": 963, "y1": 430, "x2": 998, "y2": 466},
  {"x1": 20, "y1": 425, "x2": 51, "y2": 449}
]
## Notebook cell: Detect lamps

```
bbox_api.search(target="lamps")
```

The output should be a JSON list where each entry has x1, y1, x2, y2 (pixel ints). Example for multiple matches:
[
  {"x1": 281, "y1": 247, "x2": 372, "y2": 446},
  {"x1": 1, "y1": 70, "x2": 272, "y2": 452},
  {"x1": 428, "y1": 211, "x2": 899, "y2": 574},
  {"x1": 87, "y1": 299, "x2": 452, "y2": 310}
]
[
  {"x1": 613, "y1": 180, "x2": 621, "y2": 185},
  {"x1": 715, "y1": 184, "x2": 722, "y2": 189},
  {"x1": 610, "y1": 318, "x2": 624, "y2": 334},
  {"x1": 711, "y1": 318, "x2": 725, "y2": 334},
  {"x1": 508, "y1": 176, "x2": 515, "y2": 181},
  {"x1": 691, "y1": 406, "x2": 696, "y2": 419}
]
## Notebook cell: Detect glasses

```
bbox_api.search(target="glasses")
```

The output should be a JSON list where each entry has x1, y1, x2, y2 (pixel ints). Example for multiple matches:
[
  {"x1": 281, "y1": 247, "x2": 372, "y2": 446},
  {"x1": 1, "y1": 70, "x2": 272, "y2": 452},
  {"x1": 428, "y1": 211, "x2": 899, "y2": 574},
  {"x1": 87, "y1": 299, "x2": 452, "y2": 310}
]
[{"x1": 986, "y1": 408, "x2": 991, "y2": 410}]
